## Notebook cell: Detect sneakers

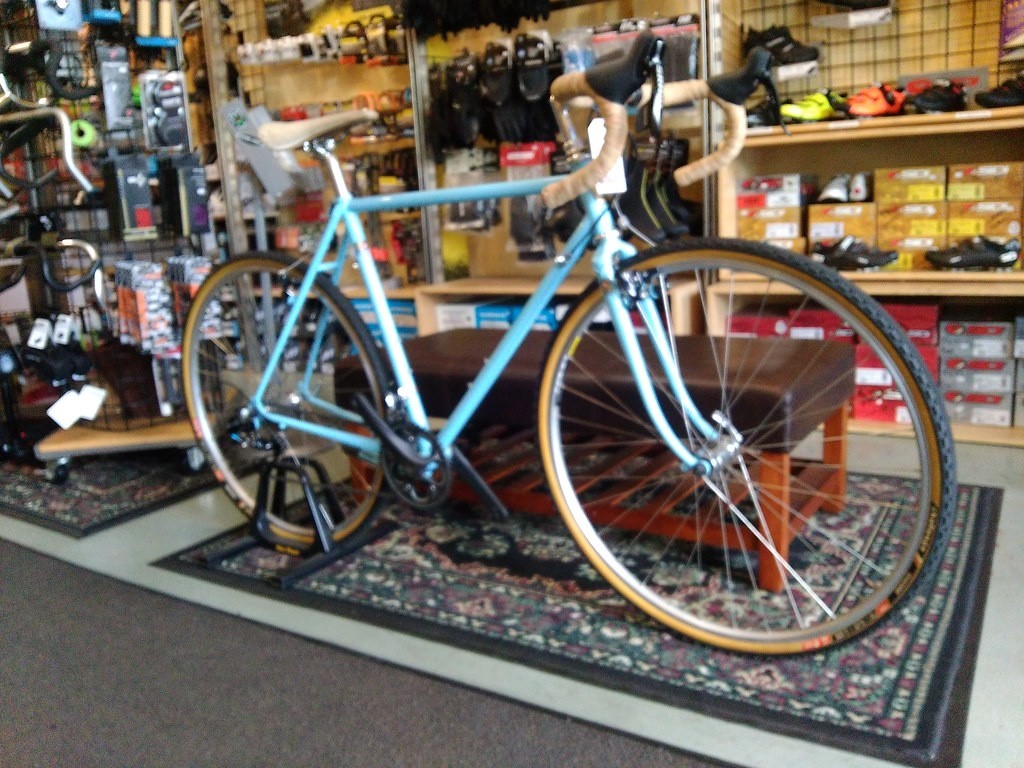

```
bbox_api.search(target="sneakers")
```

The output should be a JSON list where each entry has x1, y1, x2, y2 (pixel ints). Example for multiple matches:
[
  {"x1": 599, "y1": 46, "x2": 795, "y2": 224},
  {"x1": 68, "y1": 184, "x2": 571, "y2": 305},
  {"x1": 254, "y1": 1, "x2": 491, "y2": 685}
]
[
  {"x1": 924, "y1": 235, "x2": 1021, "y2": 273},
  {"x1": 728, "y1": 27, "x2": 1024, "y2": 123},
  {"x1": 817, "y1": 173, "x2": 851, "y2": 203},
  {"x1": 812, "y1": 234, "x2": 897, "y2": 273}
]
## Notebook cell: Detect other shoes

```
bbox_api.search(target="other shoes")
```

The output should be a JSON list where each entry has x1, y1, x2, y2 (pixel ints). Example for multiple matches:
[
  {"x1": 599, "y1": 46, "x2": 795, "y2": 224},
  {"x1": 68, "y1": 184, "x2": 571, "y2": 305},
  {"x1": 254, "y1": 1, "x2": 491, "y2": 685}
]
[{"x1": 849, "y1": 171, "x2": 871, "y2": 201}]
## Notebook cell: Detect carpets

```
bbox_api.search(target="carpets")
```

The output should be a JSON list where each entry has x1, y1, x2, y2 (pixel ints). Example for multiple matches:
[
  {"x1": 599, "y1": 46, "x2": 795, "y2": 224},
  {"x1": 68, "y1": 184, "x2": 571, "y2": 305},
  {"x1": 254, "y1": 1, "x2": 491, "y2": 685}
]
[
  {"x1": 0, "y1": 398, "x2": 337, "y2": 541},
  {"x1": 149, "y1": 426, "x2": 1007, "y2": 768}
]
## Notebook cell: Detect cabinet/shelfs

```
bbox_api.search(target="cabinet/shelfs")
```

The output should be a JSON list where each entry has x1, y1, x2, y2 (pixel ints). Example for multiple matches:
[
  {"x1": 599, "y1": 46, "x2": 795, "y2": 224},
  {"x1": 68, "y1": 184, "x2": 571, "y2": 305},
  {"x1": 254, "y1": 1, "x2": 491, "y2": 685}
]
[
  {"x1": 410, "y1": 0, "x2": 716, "y2": 337},
  {"x1": 202, "y1": 0, "x2": 435, "y2": 338},
  {"x1": 706, "y1": 0, "x2": 1024, "y2": 491}
]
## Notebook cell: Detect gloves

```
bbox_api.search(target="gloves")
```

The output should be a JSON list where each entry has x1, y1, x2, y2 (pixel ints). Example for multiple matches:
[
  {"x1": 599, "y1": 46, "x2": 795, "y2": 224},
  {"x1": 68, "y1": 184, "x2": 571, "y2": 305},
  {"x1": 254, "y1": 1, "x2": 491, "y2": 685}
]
[{"x1": 399, "y1": 0, "x2": 565, "y2": 151}]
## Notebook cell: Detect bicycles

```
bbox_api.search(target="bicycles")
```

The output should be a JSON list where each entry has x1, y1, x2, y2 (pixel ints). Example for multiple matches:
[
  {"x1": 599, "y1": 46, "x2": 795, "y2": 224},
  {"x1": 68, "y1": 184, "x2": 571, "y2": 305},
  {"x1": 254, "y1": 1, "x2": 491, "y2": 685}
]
[{"x1": 176, "y1": 25, "x2": 956, "y2": 662}]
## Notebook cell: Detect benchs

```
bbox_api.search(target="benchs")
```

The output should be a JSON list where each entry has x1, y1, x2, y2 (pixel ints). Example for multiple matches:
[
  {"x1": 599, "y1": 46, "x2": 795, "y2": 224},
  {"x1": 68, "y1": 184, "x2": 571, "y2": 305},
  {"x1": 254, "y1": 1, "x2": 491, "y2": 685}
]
[{"x1": 332, "y1": 328, "x2": 858, "y2": 595}]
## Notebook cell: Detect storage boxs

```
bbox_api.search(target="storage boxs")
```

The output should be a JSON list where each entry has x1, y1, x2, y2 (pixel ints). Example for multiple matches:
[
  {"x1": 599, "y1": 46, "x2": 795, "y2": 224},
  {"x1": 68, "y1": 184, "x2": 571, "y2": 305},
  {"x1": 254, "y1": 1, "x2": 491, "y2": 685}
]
[
  {"x1": 351, "y1": 299, "x2": 651, "y2": 356},
  {"x1": 725, "y1": 161, "x2": 1024, "y2": 430}
]
